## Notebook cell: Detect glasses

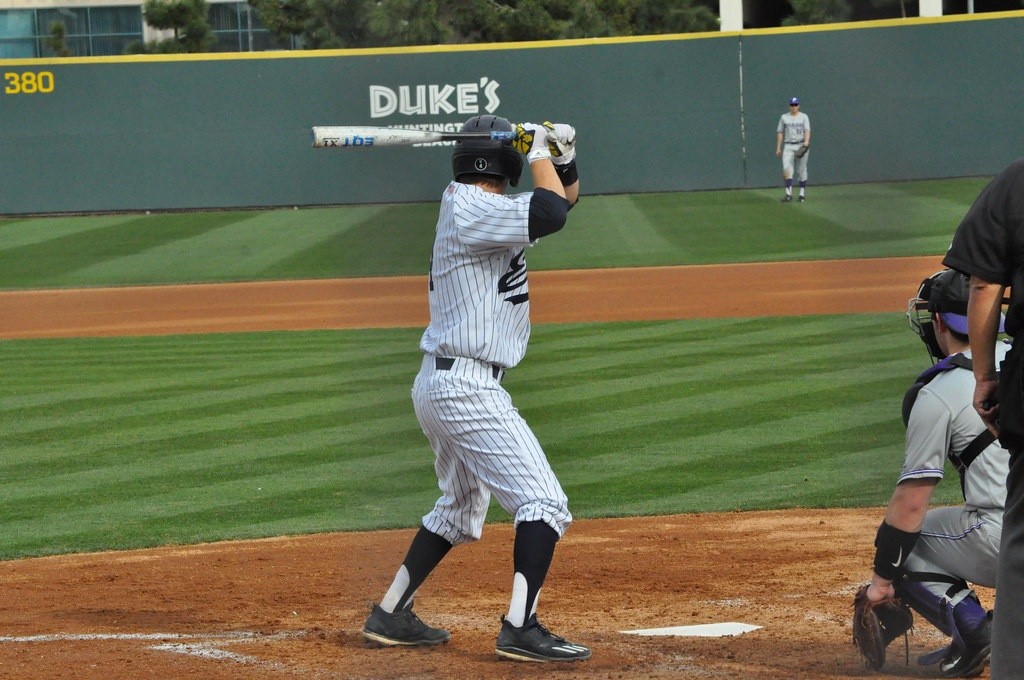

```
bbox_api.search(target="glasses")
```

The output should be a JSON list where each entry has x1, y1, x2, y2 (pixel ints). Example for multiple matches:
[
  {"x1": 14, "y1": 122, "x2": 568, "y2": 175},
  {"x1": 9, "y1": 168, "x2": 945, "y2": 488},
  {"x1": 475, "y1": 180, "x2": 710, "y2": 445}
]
[{"x1": 790, "y1": 103, "x2": 798, "y2": 106}]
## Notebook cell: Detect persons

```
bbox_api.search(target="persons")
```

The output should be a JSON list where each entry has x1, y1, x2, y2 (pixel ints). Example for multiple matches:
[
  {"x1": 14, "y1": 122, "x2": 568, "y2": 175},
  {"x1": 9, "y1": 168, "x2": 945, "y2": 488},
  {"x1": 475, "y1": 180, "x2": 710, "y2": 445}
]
[
  {"x1": 776, "y1": 97, "x2": 811, "y2": 203},
  {"x1": 942, "y1": 155, "x2": 1024, "y2": 680},
  {"x1": 362, "y1": 115, "x2": 592, "y2": 662},
  {"x1": 852, "y1": 268, "x2": 1013, "y2": 677}
]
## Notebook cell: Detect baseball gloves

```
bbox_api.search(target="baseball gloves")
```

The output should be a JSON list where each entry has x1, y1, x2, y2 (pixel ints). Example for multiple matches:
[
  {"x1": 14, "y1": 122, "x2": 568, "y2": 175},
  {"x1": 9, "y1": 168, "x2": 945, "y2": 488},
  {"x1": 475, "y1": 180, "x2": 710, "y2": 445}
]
[
  {"x1": 853, "y1": 584, "x2": 913, "y2": 670},
  {"x1": 794, "y1": 145, "x2": 808, "y2": 158}
]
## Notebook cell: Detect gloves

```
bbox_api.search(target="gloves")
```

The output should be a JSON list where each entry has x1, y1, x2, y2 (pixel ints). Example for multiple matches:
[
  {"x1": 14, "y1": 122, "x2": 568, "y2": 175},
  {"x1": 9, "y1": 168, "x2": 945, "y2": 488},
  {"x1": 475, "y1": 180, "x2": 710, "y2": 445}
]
[
  {"x1": 543, "y1": 121, "x2": 576, "y2": 165},
  {"x1": 511, "y1": 121, "x2": 551, "y2": 163}
]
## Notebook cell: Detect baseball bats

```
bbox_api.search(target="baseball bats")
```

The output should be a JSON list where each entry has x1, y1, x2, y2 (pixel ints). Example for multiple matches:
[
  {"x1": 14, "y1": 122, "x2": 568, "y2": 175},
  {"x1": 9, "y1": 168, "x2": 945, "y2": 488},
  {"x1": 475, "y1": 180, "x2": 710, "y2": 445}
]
[{"x1": 310, "y1": 124, "x2": 520, "y2": 149}]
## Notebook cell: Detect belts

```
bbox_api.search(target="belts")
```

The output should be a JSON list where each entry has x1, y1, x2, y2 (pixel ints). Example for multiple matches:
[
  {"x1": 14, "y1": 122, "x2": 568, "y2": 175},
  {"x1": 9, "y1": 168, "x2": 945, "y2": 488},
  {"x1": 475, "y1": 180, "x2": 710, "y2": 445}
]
[
  {"x1": 785, "y1": 141, "x2": 804, "y2": 144},
  {"x1": 435, "y1": 357, "x2": 506, "y2": 383}
]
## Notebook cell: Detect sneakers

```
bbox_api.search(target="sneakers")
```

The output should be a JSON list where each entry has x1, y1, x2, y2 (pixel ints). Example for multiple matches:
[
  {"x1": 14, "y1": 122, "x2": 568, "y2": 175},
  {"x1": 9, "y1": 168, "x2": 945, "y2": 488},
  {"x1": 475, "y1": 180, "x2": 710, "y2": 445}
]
[
  {"x1": 798, "y1": 195, "x2": 804, "y2": 202},
  {"x1": 939, "y1": 608, "x2": 994, "y2": 678},
  {"x1": 780, "y1": 195, "x2": 793, "y2": 202},
  {"x1": 495, "y1": 612, "x2": 592, "y2": 663},
  {"x1": 362, "y1": 600, "x2": 452, "y2": 647},
  {"x1": 916, "y1": 643, "x2": 952, "y2": 675}
]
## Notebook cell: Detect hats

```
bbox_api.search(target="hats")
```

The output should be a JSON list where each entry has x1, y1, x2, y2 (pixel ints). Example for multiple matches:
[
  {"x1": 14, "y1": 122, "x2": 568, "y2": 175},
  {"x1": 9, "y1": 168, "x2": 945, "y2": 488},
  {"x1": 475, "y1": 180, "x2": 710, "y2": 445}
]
[
  {"x1": 936, "y1": 269, "x2": 1006, "y2": 334},
  {"x1": 789, "y1": 96, "x2": 799, "y2": 105}
]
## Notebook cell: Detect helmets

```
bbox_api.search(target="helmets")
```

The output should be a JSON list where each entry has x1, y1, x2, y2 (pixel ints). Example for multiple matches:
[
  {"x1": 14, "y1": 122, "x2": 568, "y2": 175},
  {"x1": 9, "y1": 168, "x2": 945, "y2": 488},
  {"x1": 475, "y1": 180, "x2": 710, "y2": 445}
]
[{"x1": 452, "y1": 115, "x2": 524, "y2": 188}]
